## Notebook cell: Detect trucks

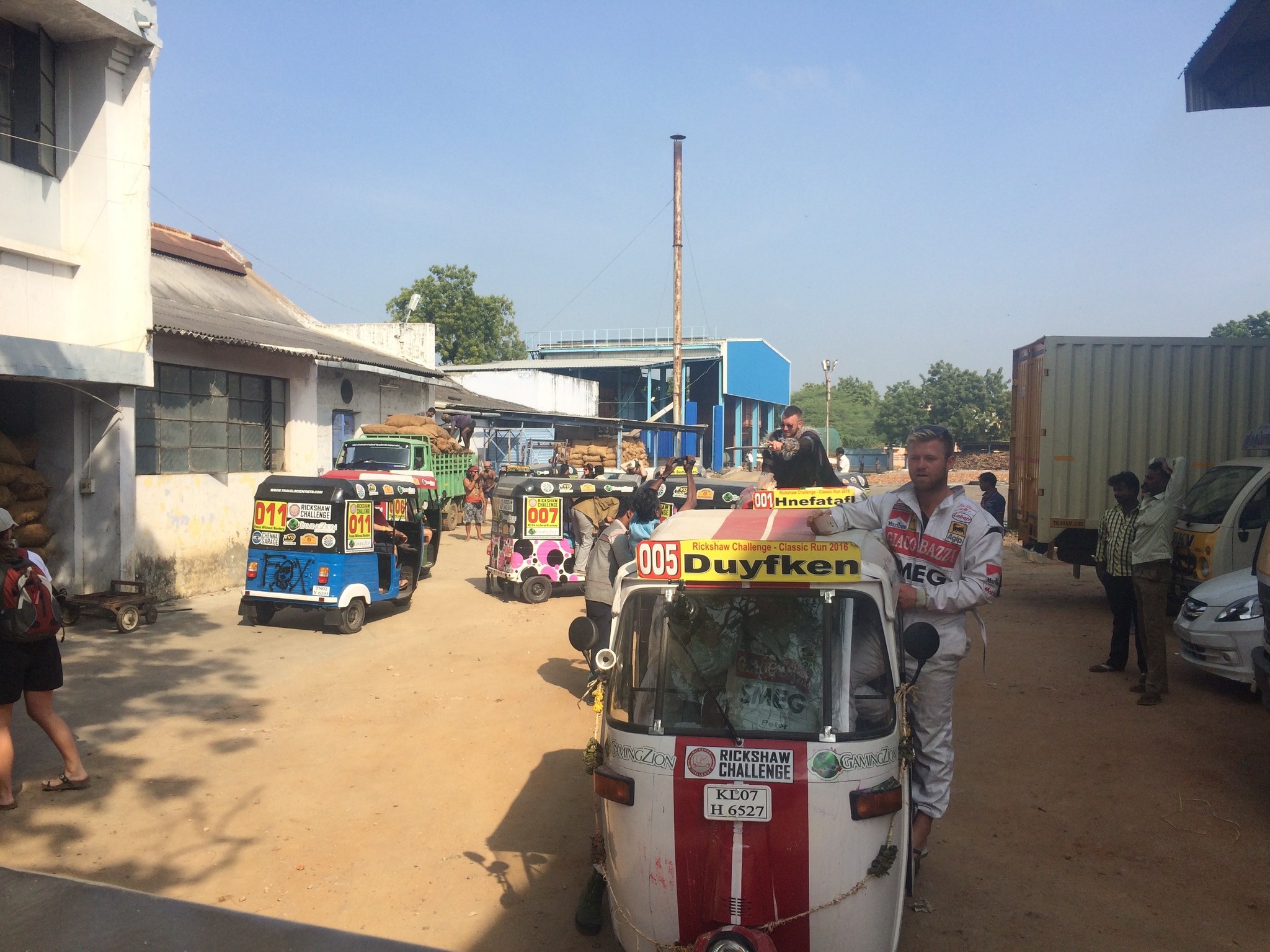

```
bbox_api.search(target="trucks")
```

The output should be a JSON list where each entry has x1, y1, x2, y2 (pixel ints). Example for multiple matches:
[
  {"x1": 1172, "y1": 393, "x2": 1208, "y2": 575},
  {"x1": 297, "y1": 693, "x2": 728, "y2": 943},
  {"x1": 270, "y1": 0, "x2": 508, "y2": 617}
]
[{"x1": 332, "y1": 433, "x2": 477, "y2": 532}]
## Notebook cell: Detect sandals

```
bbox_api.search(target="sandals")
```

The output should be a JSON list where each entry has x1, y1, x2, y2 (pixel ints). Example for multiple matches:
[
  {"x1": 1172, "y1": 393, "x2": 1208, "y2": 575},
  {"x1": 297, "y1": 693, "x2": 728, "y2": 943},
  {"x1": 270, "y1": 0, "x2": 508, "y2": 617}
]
[{"x1": 378, "y1": 589, "x2": 388, "y2": 594}]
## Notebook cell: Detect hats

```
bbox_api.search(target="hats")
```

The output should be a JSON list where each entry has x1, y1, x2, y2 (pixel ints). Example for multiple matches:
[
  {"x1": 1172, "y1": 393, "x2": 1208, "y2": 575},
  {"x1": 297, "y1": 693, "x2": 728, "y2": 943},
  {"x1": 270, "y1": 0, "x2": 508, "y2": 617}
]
[
  {"x1": 0, "y1": 508, "x2": 19, "y2": 532},
  {"x1": 628, "y1": 461, "x2": 639, "y2": 470}
]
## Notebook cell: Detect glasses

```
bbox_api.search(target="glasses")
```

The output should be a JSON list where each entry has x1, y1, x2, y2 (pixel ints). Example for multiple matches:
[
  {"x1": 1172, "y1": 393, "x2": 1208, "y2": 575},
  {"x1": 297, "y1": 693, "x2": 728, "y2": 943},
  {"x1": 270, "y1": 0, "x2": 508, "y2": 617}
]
[
  {"x1": 583, "y1": 469, "x2": 588, "y2": 471},
  {"x1": 779, "y1": 422, "x2": 797, "y2": 429},
  {"x1": 471, "y1": 470, "x2": 479, "y2": 473}
]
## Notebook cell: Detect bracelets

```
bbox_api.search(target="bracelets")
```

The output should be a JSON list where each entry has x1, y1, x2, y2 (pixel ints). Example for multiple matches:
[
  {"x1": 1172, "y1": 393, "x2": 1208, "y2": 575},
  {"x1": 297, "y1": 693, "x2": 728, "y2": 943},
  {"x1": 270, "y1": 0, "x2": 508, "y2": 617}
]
[{"x1": 472, "y1": 479, "x2": 476, "y2": 482}]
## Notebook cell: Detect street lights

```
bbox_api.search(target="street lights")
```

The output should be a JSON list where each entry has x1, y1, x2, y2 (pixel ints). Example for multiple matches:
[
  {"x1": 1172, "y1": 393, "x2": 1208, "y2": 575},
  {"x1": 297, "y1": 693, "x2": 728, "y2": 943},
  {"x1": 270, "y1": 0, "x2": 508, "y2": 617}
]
[{"x1": 821, "y1": 358, "x2": 839, "y2": 458}]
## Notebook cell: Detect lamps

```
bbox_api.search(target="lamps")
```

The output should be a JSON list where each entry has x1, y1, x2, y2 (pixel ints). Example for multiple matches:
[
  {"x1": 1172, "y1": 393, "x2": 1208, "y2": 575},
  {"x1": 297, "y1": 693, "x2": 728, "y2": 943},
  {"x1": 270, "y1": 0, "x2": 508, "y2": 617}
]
[
  {"x1": 379, "y1": 382, "x2": 400, "y2": 389},
  {"x1": 405, "y1": 294, "x2": 421, "y2": 322}
]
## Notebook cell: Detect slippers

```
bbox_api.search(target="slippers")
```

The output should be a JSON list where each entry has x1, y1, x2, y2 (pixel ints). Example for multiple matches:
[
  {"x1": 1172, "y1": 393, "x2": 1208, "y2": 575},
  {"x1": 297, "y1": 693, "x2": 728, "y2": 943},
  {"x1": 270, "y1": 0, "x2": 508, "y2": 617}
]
[
  {"x1": 912, "y1": 848, "x2": 929, "y2": 860},
  {"x1": 0, "y1": 800, "x2": 18, "y2": 810},
  {"x1": 42, "y1": 773, "x2": 92, "y2": 791},
  {"x1": 399, "y1": 579, "x2": 408, "y2": 590}
]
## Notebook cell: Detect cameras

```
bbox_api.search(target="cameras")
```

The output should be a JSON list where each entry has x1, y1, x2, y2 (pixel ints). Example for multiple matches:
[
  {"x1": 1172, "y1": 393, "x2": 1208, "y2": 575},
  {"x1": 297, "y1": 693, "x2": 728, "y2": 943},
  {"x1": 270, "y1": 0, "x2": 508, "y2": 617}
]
[{"x1": 675, "y1": 456, "x2": 689, "y2": 466}]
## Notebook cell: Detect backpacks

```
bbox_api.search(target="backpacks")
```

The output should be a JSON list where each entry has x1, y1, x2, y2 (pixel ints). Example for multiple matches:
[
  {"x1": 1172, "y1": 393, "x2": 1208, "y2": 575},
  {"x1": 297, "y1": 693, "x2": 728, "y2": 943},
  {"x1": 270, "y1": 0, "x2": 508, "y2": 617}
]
[{"x1": 0, "y1": 547, "x2": 65, "y2": 642}]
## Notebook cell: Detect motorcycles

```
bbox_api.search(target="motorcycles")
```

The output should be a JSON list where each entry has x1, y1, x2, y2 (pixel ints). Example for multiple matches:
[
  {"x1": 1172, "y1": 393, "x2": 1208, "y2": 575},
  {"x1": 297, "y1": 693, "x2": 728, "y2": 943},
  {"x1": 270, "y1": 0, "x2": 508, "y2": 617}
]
[
  {"x1": 484, "y1": 462, "x2": 871, "y2": 605},
  {"x1": 319, "y1": 469, "x2": 448, "y2": 577},
  {"x1": 237, "y1": 474, "x2": 425, "y2": 635},
  {"x1": 1169, "y1": 455, "x2": 1270, "y2": 679},
  {"x1": 568, "y1": 504, "x2": 943, "y2": 952}
]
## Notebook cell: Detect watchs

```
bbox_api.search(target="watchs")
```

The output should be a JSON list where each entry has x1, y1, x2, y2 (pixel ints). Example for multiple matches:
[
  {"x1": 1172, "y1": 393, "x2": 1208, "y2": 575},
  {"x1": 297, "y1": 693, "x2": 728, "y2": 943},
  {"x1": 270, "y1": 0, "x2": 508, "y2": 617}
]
[{"x1": 659, "y1": 474, "x2": 666, "y2": 481}]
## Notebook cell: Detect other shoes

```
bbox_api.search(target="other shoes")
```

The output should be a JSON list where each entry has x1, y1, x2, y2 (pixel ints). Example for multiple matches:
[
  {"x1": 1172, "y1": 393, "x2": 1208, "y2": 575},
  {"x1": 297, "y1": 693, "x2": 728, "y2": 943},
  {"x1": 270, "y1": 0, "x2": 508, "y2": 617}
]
[
  {"x1": 573, "y1": 569, "x2": 586, "y2": 576},
  {"x1": 1129, "y1": 683, "x2": 1145, "y2": 693},
  {"x1": 587, "y1": 700, "x2": 594, "y2": 706},
  {"x1": 1139, "y1": 673, "x2": 1146, "y2": 682},
  {"x1": 1137, "y1": 690, "x2": 1163, "y2": 705},
  {"x1": 1089, "y1": 663, "x2": 1113, "y2": 672}
]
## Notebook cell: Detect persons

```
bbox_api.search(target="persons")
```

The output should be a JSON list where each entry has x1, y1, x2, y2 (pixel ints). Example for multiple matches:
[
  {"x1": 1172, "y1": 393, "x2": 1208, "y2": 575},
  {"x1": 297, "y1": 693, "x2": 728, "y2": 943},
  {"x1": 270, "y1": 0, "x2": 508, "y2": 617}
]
[
  {"x1": 833, "y1": 447, "x2": 850, "y2": 473},
  {"x1": 756, "y1": 406, "x2": 846, "y2": 487},
  {"x1": 978, "y1": 472, "x2": 1006, "y2": 537},
  {"x1": 462, "y1": 465, "x2": 486, "y2": 541},
  {"x1": 413, "y1": 407, "x2": 436, "y2": 418},
  {"x1": 441, "y1": 414, "x2": 476, "y2": 451},
  {"x1": 0, "y1": 507, "x2": 88, "y2": 805},
  {"x1": 859, "y1": 456, "x2": 864, "y2": 473},
  {"x1": 584, "y1": 495, "x2": 633, "y2": 707},
  {"x1": 553, "y1": 455, "x2": 698, "y2": 574},
  {"x1": 1089, "y1": 471, "x2": 1147, "y2": 682},
  {"x1": 373, "y1": 497, "x2": 433, "y2": 592},
  {"x1": 875, "y1": 457, "x2": 879, "y2": 472},
  {"x1": 478, "y1": 461, "x2": 496, "y2": 523},
  {"x1": 1132, "y1": 456, "x2": 1189, "y2": 706},
  {"x1": 808, "y1": 425, "x2": 1006, "y2": 874}
]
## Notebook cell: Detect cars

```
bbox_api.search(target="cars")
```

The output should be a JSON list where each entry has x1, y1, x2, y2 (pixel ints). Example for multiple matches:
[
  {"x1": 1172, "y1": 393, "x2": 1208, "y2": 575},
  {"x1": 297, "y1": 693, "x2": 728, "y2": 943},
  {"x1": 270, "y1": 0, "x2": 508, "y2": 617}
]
[{"x1": 1172, "y1": 567, "x2": 1266, "y2": 683}]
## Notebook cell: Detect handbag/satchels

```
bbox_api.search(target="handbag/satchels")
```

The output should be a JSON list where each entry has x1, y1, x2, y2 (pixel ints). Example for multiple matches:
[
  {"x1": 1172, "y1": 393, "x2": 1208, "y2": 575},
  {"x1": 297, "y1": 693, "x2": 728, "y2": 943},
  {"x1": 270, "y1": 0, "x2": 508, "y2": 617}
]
[{"x1": 472, "y1": 501, "x2": 482, "y2": 510}]
